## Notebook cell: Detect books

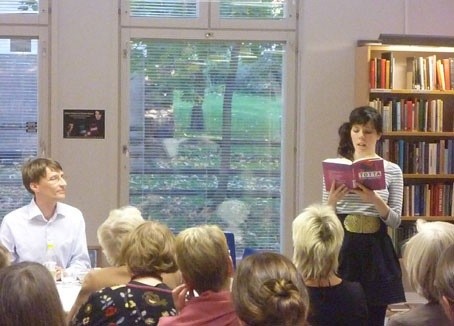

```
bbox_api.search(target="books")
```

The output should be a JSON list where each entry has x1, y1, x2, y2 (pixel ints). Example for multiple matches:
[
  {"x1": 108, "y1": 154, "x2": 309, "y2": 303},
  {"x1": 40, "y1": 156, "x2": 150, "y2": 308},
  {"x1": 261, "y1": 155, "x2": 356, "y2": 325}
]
[
  {"x1": 376, "y1": 137, "x2": 454, "y2": 174},
  {"x1": 401, "y1": 182, "x2": 454, "y2": 216},
  {"x1": 323, "y1": 158, "x2": 386, "y2": 194},
  {"x1": 406, "y1": 55, "x2": 454, "y2": 90},
  {"x1": 395, "y1": 225, "x2": 417, "y2": 258},
  {"x1": 358, "y1": 40, "x2": 383, "y2": 46},
  {"x1": 369, "y1": 98, "x2": 443, "y2": 132},
  {"x1": 370, "y1": 52, "x2": 395, "y2": 89}
]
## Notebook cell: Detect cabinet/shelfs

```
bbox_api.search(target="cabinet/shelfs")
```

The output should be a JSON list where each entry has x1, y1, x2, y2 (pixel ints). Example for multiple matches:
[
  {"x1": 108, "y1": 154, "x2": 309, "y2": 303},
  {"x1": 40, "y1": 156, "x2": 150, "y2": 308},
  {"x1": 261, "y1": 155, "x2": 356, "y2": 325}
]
[{"x1": 354, "y1": 45, "x2": 454, "y2": 253}]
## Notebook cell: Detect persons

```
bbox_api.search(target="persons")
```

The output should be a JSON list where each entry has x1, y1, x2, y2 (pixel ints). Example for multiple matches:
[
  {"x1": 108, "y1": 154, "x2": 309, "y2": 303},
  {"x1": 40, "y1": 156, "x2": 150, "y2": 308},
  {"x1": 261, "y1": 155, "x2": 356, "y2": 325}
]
[
  {"x1": 67, "y1": 206, "x2": 184, "y2": 326},
  {"x1": 0, "y1": 158, "x2": 91, "y2": 284},
  {"x1": 68, "y1": 220, "x2": 180, "y2": 326},
  {"x1": 65, "y1": 110, "x2": 104, "y2": 139},
  {"x1": 386, "y1": 219, "x2": 454, "y2": 326},
  {"x1": 323, "y1": 106, "x2": 407, "y2": 326},
  {"x1": 235, "y1": 253, "x2": 309, "y2": 326},
  {"x1": 291, "y1": 205, "x2": 369, "y2": 326},
  {"x1": 0, "y1": 242, "x2": 67, "y2": 326},
  {"x1": 433, "y1": 245, "x2": 454, "y2": 322},
  {"x1": 157, "y1": 224, "x2": 247, "y2": 326}
]
